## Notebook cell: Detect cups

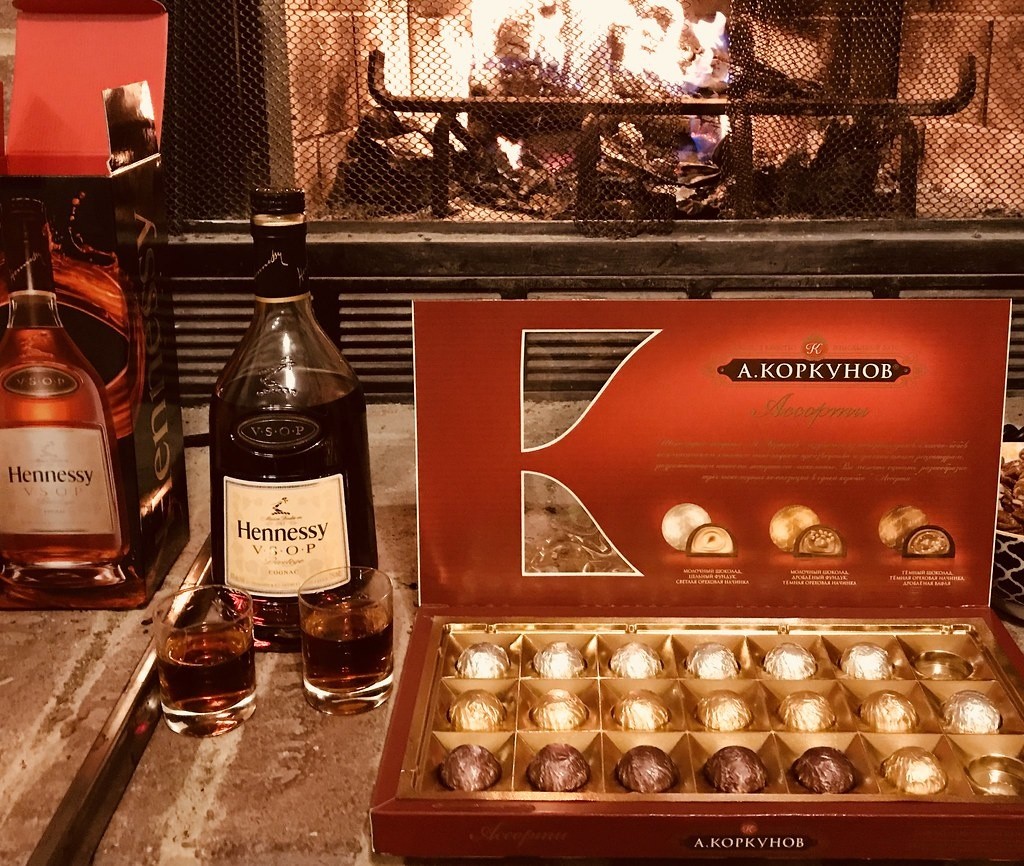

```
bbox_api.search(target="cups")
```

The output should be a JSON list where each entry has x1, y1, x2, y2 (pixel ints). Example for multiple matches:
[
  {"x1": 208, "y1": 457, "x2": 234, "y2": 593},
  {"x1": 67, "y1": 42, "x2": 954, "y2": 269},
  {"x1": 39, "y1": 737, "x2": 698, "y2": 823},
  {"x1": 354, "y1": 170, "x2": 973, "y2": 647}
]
[
  {"x1": 152, "y1": 583, "x2": 258, "y2": 737},
  {"x1": 299, "y1": 566, "x2": 393, "y2": 715}
]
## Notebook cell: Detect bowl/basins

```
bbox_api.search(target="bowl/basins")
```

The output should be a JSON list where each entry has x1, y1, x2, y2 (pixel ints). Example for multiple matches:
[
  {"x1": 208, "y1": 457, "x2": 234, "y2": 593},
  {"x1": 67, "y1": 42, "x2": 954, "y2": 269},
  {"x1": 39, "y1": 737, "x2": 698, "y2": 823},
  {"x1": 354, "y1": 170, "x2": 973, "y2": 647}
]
[{"x1": 992, "y1": 441, "x2": 1024, "y2": 621}]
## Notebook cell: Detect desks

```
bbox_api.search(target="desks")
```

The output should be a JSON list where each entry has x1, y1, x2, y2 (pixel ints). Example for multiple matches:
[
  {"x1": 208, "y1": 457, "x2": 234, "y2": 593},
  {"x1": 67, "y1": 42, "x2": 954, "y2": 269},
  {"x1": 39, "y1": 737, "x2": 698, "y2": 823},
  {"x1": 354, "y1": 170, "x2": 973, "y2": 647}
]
[{"x1": 0, "y1": 402, "x2": 1024, "y2": 864}]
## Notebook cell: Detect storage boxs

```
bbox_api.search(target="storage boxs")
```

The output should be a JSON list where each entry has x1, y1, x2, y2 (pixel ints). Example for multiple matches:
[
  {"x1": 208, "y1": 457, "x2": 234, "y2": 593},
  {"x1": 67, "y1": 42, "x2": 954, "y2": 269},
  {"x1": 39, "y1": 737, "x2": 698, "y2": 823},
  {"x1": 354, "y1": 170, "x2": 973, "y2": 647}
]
[
  {"x1": 0, "y1": 0, "x2": 189, "y2": 611},
  {"x1": 369, "y1": 296, "x2": 1024, "y2": 862}
]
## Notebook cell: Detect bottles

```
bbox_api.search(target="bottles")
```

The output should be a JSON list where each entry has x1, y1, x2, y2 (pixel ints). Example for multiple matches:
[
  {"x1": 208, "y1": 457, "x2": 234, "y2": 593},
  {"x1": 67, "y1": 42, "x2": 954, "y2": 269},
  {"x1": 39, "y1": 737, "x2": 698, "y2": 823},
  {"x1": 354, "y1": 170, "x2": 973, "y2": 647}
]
[
  {"x1": 209, "y1": 189, "x2": 380, "y2": 651},
  {"x1": 2, "y1": 196, "x2": 132, "y2": 591}
]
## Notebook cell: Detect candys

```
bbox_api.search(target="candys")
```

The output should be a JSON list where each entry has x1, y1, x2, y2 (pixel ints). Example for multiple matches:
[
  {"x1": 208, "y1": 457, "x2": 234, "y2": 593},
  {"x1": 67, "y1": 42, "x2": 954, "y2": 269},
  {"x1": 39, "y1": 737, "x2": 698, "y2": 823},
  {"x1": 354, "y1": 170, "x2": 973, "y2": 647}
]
[
  {"x1": 436, "y1": 639, "x2": 1003, "y2": 794},
  {"x1": 661, "y1": 502, "x2": 957, "y2": 560}
]
